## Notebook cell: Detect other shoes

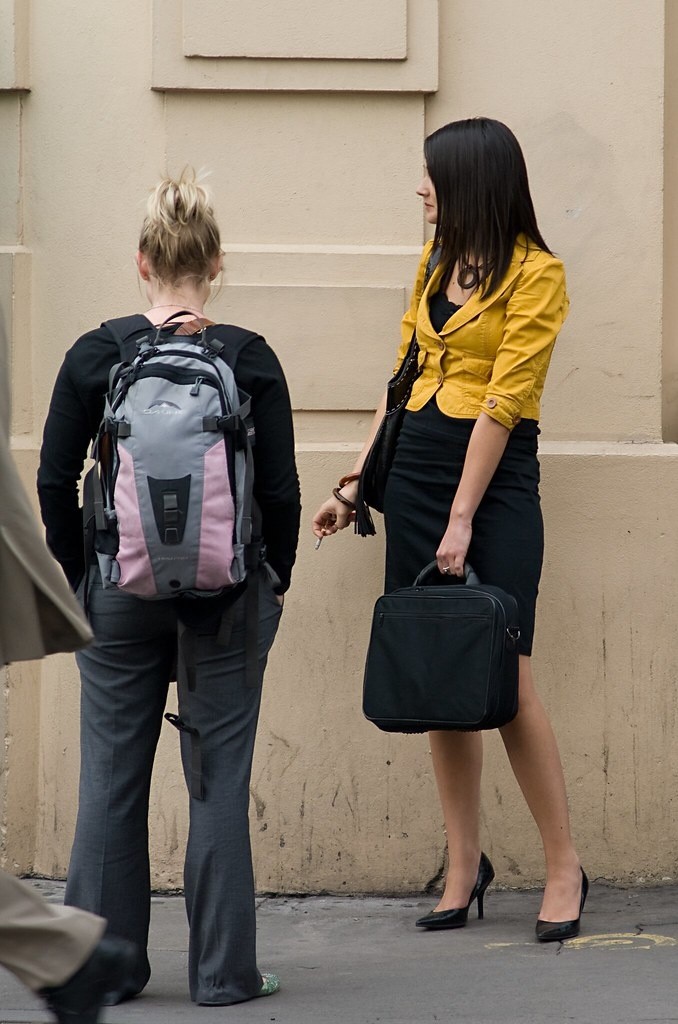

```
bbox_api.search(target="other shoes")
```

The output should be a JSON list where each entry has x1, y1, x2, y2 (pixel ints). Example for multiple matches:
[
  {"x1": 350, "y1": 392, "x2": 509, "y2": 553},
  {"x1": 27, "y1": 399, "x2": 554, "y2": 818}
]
[
  {"x1": 38, "y1": 934, "x2": 136, "y2": 1024},
  {"x1": 199, "y1": 973, "x2": 280, "y2": 1004}
]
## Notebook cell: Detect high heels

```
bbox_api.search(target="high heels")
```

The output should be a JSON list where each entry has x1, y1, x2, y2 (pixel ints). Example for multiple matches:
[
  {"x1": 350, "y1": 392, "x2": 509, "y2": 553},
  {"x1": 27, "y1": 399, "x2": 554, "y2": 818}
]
[
  {"x1": 416, "y1": 851, "x2": 495, "y2": 927},
  {"x1": 535, "y1": 865, "x2": 589, "y2": 939}
]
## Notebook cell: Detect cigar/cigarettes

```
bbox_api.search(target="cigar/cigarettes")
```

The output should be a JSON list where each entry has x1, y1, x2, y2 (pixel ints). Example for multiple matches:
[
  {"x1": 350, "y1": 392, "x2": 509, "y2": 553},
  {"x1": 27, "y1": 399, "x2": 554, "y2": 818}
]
[{"x1": 314, "y1": 529, "x2": 326, "y2": 550}]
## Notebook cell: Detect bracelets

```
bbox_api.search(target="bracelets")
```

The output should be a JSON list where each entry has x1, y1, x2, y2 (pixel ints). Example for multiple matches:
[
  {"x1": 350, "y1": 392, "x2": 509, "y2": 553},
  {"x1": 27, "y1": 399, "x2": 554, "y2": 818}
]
[
  {"x1": 333, "y1": 488, "x2": 355, "y2": 509},
  {"x1": 339, "y1": 472, "x2": 361, "y2": 486}
]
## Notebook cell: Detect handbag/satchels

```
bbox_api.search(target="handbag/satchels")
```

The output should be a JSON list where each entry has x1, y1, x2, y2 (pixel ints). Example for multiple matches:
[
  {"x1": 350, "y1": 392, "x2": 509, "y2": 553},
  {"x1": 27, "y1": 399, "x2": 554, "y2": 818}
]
[
  {"x1": 355, "y1": 330, "x2": 423, "y2": 537},
  {"x1": 363, "y1": 559, "x2": 521, "y2": 734}
]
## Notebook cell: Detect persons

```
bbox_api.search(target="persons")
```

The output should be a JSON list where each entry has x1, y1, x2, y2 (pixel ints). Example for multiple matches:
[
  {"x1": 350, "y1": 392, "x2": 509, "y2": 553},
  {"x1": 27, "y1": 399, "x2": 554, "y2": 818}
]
[
  {"x1": 312, "y1": 118, "x2": 590, "y2": 943},
  {"x1": 33, "y1": 164, "x2": 300, "y2": 1006},
  {"x1": 0, "y1": 252, "x2": 135, "y2": 1024}
]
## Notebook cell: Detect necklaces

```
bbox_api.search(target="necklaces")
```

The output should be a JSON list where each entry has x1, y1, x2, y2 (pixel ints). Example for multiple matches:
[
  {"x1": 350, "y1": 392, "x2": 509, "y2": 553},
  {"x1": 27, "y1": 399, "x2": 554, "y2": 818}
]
[
  {"x1": 456, "y1": 259, "x2": 493, "y2": 289},
  {"x1": 145, "y1": 305, "x2": 205, "y2": 317}
]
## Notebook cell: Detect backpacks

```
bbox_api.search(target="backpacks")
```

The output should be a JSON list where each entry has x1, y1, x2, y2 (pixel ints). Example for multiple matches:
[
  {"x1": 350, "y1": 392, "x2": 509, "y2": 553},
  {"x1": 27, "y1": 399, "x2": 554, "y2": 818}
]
[{"x1": 85, "y1": 314, "x2": 268, "y2": 690}]
